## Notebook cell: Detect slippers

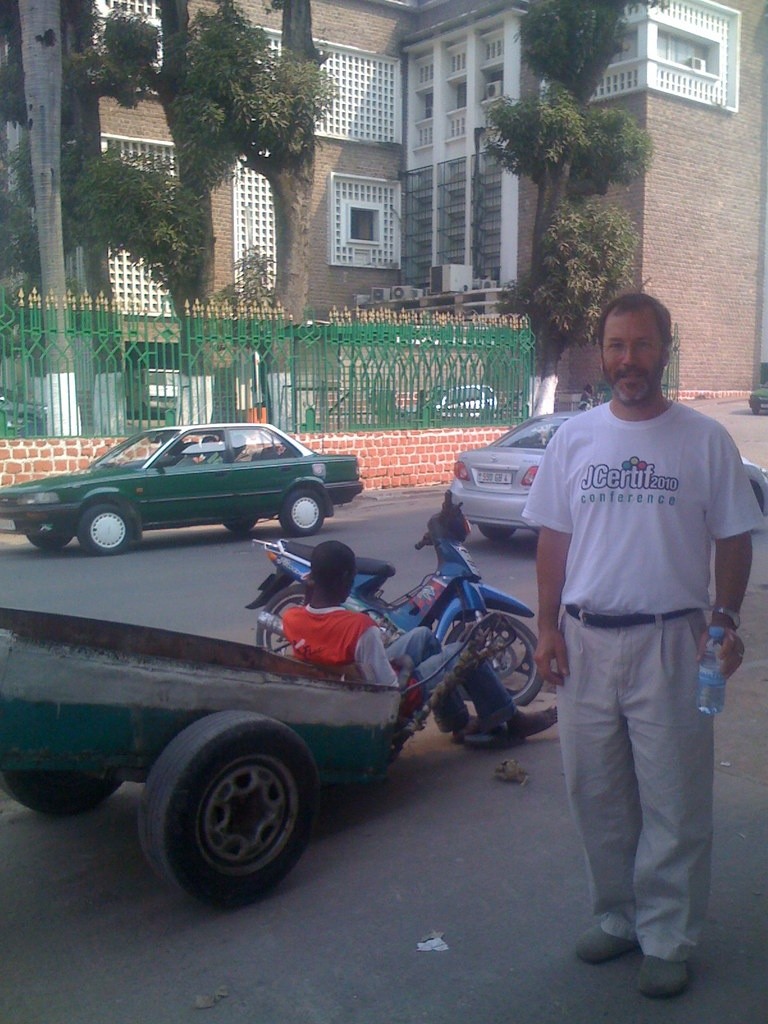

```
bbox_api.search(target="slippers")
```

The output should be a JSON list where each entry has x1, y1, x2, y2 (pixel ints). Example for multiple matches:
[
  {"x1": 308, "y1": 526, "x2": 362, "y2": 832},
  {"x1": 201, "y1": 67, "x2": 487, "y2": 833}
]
[
  {"x1": 463, "y1": 724, "x2": 525, "y2": 748},
  {"x1": 456, "y1": 719, "x2": 479, "y2": 744}
]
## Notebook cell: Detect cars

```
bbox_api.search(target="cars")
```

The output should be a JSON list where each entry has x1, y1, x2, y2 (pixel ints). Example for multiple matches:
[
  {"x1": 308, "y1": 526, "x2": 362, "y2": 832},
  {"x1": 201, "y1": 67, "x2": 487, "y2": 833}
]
[
  {"x1": 436, "y1": 385, "x2": 498, "y2": 418},
  {"x1": 749, "y1": 380, "x2": 768, "y2": 414},
  {"x1": 0, "y1": 423, "x2": 363, "y2": 556},
  {"x1": 148, "y1": 368, "x2": 180, "y2": 419},
  {"x1": 450, "y1": 412, "x2": 768, "y2": 539}
]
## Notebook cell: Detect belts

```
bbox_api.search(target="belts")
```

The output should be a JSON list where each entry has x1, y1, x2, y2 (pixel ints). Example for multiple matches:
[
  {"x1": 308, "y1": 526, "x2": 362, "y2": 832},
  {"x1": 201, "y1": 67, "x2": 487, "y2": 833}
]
[{"x1": 566, "y1": 604, "x2": 696, "y2": 628}]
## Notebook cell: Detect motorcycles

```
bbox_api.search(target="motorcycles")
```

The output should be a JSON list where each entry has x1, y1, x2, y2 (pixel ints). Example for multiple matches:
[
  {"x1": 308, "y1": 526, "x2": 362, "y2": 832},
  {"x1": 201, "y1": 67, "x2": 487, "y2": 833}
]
[{"x1": 244, "y1": 489, "x2": 544, "y2": 706}]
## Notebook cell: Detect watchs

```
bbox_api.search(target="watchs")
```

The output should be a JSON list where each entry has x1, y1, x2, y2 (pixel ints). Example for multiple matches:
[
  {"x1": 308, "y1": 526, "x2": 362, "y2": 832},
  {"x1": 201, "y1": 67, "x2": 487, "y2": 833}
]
[{"x1": 711, "y1": 606, "x2": 741, "y2": 629}]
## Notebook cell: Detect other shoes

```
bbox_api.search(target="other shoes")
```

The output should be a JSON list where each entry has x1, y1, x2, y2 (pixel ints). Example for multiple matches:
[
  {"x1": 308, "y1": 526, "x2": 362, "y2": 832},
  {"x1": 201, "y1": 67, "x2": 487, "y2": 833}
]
[
  {"x1": 637, "y1": 956, "x2": 694, "y2": 997},
  {"x1": 574, "y1": 924, "x2": 634, "y2": 965}
]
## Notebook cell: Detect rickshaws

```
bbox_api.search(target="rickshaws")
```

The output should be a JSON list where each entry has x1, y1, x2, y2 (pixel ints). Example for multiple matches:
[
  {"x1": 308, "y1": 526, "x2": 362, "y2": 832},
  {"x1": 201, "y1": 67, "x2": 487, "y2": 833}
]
[{"x1": 0, "y1": 607, "x2": 516, "y2": 902}]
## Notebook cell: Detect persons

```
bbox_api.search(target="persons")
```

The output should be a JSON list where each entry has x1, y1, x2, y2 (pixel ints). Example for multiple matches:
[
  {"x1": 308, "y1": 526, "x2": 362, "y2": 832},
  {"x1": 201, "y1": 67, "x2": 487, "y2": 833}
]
[
  {"x1": 193, "y1": 436, "x2": 224, "y2": 464},
  {"x1": 522, "y1": 293, "x2": 765, "y2": 998},
  {"x1": 283, "y1": 540, "x2": 557, "y2": 746},
  {"x1": 232, "y1": 434, "x2": 252, "y2": 462}
]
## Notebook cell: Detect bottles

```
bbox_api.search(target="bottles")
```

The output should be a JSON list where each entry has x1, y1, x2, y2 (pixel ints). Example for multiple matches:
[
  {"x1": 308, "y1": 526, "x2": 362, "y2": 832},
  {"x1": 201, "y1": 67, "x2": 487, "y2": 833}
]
[{"x1": 696, "y1": 625, "x2": 726, "y2": 715}]
